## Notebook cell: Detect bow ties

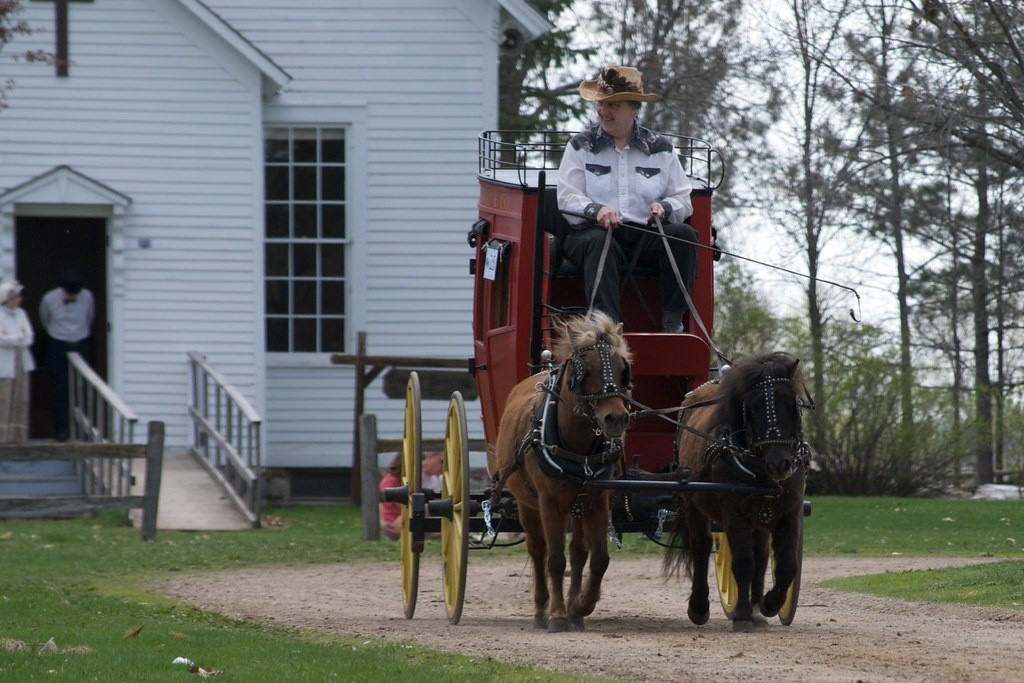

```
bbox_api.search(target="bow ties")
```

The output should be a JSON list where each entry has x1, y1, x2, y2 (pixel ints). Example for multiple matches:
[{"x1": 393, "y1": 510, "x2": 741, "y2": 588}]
[{"x1": 66, "y1": 299, "x2": 75, "y2": 303}]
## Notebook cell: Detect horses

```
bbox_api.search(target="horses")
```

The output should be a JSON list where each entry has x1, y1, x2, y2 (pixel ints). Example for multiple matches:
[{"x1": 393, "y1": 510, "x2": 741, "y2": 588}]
[
  {"x1": 495, "y1": 312, "x2": 632, "y2": 634},
  {"x1": 676, "y1": 355, "x2": 805, "y2": 632}
]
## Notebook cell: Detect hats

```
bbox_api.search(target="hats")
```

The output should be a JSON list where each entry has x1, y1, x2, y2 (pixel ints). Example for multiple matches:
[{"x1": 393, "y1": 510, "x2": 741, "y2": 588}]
[
  {"x1": 63, "y1": 274, "x2": 82, "y2": 293},
  {"x1": 579, "y1": 65, "x2": 659, "y2": 101},
  {"x1": 0, "y1": 281, "x2": 24, "y2": 304}
]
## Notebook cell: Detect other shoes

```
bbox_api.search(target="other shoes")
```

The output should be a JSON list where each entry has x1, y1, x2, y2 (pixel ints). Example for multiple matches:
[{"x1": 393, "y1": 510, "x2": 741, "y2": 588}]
[{"x1": 661, "y1": 308, "x2": 684, "y2": 334}]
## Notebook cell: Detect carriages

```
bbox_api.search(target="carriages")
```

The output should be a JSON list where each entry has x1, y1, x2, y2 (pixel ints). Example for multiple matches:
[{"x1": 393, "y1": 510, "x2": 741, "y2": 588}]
[{"x1": 379, "y1": 130, "x2": 815, "y2": 632}]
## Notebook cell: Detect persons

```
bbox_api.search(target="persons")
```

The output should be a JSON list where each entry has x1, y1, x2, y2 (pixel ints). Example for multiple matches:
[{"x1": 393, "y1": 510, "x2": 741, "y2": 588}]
[
  {"x1": 555, "y1": 65, "x2": 699, "y2": 334},
  {"x1": 37, "y1": 272, "x2": 95, "y2": 442},
  {"x1": 0, "y1": 280, "x2": 36, "y2": 447}
]
